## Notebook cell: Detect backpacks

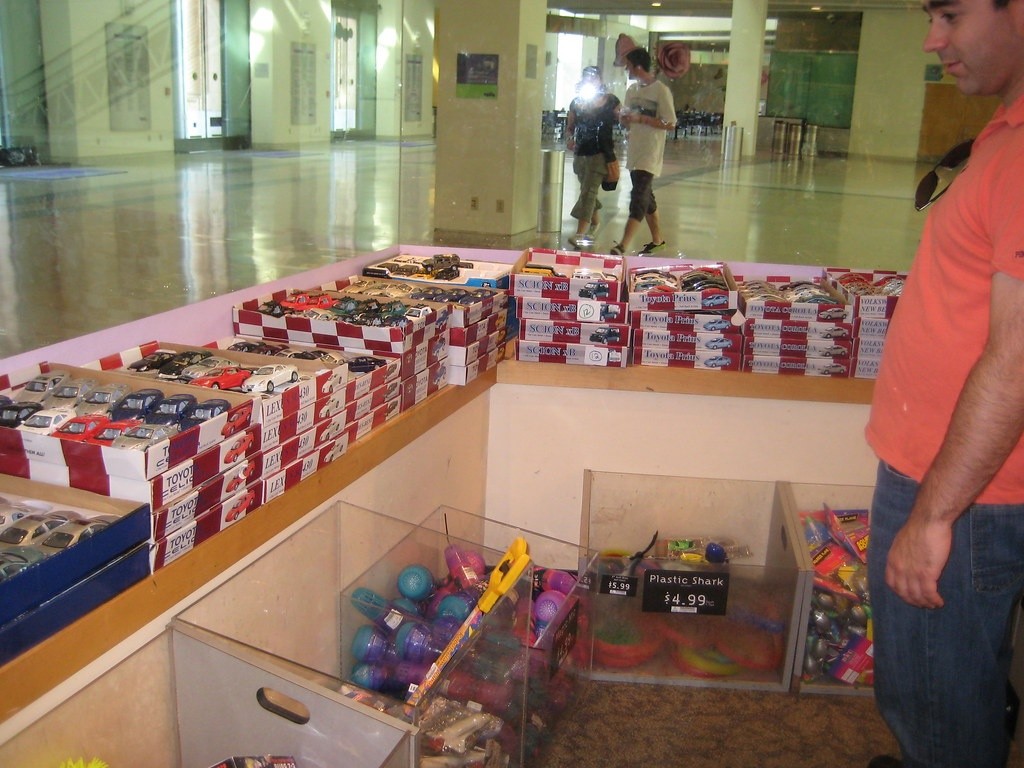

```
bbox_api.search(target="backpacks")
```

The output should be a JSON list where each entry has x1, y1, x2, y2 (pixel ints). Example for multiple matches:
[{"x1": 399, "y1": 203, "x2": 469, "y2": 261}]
[{"x1": 573, "y1": 108, "x2": 612, "y2": 155}]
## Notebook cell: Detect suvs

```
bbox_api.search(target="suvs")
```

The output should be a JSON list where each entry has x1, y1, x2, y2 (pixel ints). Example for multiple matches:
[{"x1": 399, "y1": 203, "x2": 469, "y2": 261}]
[{"x1": 403, "y1": 304, "x2": 432, "y2": 321}]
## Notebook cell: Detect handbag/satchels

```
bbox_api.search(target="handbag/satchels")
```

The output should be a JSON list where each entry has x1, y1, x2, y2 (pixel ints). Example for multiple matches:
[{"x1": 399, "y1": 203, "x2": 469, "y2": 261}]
[{"x1": 602, "y1": 151, "x2": 618, "y2": 192}]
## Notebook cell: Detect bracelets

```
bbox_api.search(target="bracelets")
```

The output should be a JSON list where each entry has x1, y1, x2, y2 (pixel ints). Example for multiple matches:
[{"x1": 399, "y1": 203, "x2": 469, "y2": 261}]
[{"x1": 640, "y1": 114, "x2": 642, "y2": 123}]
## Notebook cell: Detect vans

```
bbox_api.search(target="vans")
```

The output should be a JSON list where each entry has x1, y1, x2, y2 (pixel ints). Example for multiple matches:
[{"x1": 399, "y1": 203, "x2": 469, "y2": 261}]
[
  {"x1": 521, "y1": 262, "x2": 609, "y2": 280},
  {"x1": 578, "y1": 282, "x2": 620, "y2": 344}
]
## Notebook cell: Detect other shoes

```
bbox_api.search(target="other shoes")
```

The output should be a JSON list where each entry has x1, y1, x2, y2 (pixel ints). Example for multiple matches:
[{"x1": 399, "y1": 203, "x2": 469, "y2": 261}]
[
  {"x1": 568, "y1": 234, "x2": 584, "y2": 251},
  {"x1": 583, "y1": 224, "x2": 600, "y2": 240}
]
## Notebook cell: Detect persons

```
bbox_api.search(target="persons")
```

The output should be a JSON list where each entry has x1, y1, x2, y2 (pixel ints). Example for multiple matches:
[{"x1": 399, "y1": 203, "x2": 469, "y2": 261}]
[
  {"x1": 864, "y1": 0, "x2": 1024, "y2": 768},
  {"x1": 677, "y1": 103, "x2": 705, "y2": 138},
  {"x1": 566, "y1": 66, "x2": 629, "y2": 247},
  {"x1": 611, "y1": 49, "x2": 677, "y2": 257}
]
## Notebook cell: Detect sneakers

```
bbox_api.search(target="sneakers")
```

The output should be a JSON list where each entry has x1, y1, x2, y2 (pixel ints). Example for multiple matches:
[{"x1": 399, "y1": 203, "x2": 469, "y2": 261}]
[
  {"x1": 638, "y1": 241, "x2": 666, "y2": 257},
  {"x1": 610, "y1": 240, "x2": 625, "y2": 255}
]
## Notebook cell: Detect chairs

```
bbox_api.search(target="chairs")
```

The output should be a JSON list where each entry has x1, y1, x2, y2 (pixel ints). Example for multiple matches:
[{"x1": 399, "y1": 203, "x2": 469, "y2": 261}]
[{"x1": 542, "y1": 110, "x2": 725, "y2": 141}]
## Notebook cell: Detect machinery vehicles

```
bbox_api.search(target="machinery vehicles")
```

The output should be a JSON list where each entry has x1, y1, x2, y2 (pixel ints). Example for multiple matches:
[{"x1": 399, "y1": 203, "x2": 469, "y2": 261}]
[{"x1": 378, "y1": 254, "x2": 473, "y2": 283}]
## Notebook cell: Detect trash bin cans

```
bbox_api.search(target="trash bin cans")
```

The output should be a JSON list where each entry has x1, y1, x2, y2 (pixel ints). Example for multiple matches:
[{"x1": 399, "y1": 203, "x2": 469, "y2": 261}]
[
  {"x1": 771, "y1": 121, "x2": 788, "y2": 154},
  {"x1": 802, "y1": 124, "x2": 818, "y2": 156},
  {"x1": 536, "y1": 150, "x2": 566, "y2": 234},
  {"x1": 787, "y1": 123, "x2": 802, "y2": 155},
  {"x1": 721, "y1": 126, "x2": 744, "y2": 162}
]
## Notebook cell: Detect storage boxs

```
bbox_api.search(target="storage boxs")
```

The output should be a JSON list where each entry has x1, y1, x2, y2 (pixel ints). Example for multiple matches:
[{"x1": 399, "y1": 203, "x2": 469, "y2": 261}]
[
  {"x1": 743, "y1": 337, "x2": 853, "y2": 359},
  {"x1": 632, "y1": 328, "x2": 743, "y2": 354},
  {"x1": 628, "y1": 261, "x2": 739, "y2": 311},
  {"x1": 338, "y1": 505, "x2": 600, "y2": 768},
  {"x1": 742, "y1": 355, "x2": 850, "y2": 378},
  {"x1": 513, "y1": 338, "x2": 631, "y2": 368},
  {"x1": 518, "y1": 317, "x2": 633, "y2": 349},
  {"x1": 0, "y1": 251, "x2": 519, "y2": 667},
  {"x1": 824, "y1": 267, "x2": 908, "y2": 379},
  {"x1": 509, "y1": 246, "x2": 627, "y2": 302},
  {"x1": 163, "y1": 500, "x2": 534, "y2": 768},
  {"x1": 784, "y1": 481, "x2": 876, "y2": 697},
  {"x1": 734, "y1": 274, "x2": 853, "y2": 324},
  {"x1": 632, "y1": 347, "x2": 741, "y2": 373},
  {"x1": 631, "y1": 310, "x2": 740, "y2": 334},
  {"x1": 515, "y1": 295, "x2": 629, "y2": 325},
  {"x1": 741, "y1": 318, "x2": 853, "y2": 341},
  {"x1": 575, "y1": 469, "x2": 805, "y2": 693}
]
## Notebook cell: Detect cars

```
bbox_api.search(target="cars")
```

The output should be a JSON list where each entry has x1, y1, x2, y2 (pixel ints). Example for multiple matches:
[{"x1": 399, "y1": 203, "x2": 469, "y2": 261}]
[
  {"x1": 189, "y1": 366, "x2": 255, "y2": 390},
  {"x1": 385, "y1": 363, "x2": 400, "y2": 415},
  {"x1": 226, "y1": 342, "x2": 346, "y2": 365},
  {"x1": 839, "y1": 273, "x2": 905, "y2": 298},
  {"x1": 181, "y1": 356, "x2": 241, "y2": 382},
  {"x1": 156, "y1": 350, "x2": 215, "y2": 382},
  {"x1": 178, "y1": 398, "x2": 232, "y2": 431},
  {"x1": 256, "y1": 279, "x2": 422, "y2": 327},
  {"x1": 432, "y1": 288, "x2": 470, "y2": 303},
  {"x1": 458, "y1": 289, "x2": 499, "y2": 308},
  {"x1": 0, "y1": 369, "x2": 197, "y2": 454},
  {"x1": 126, "y1": 351, "x2": 178, "y2": 373},
  {"x1": 0, "y1": 495, "x2": 120, "y2": 587},
  {"x1": 318, "y1": 374, "x2": 345, "y2": 464},
  {"x1": 410, "y1": 286, "x2": 445, "y2": 305},
  {"x1": 739, "y1": 279, "x2": 839, "y2": 305},
  {"x1": 631, "y1": 268, "x2": 729, "y2": 292},
  {"x1": 242, "y1": 363, "x2": 299, "y2": 395},
  {"x1": 701, "y1": 294, "x2": 733, "y2": 368},
  {"x1": 493, "y1": 294, "x2": 507, "y2": 362},
  {"x1": 221, "y1": 406, "x2": 256, "y2": 522},
  {"x1": 818, "y1": 308, "x2": 850, "y2": 375},
  {"x1": 347, "y1": 355, "x2": 387, "y2": 373}
]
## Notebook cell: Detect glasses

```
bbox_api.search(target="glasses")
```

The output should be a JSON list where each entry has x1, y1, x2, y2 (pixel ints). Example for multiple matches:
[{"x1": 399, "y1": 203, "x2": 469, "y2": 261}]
[
  {"x1": 915, "y1": 139, "x2": 976, "y2": 212},
  {"x1": 583, "y1": 72, "x2": 599, "y2": 79}
]
